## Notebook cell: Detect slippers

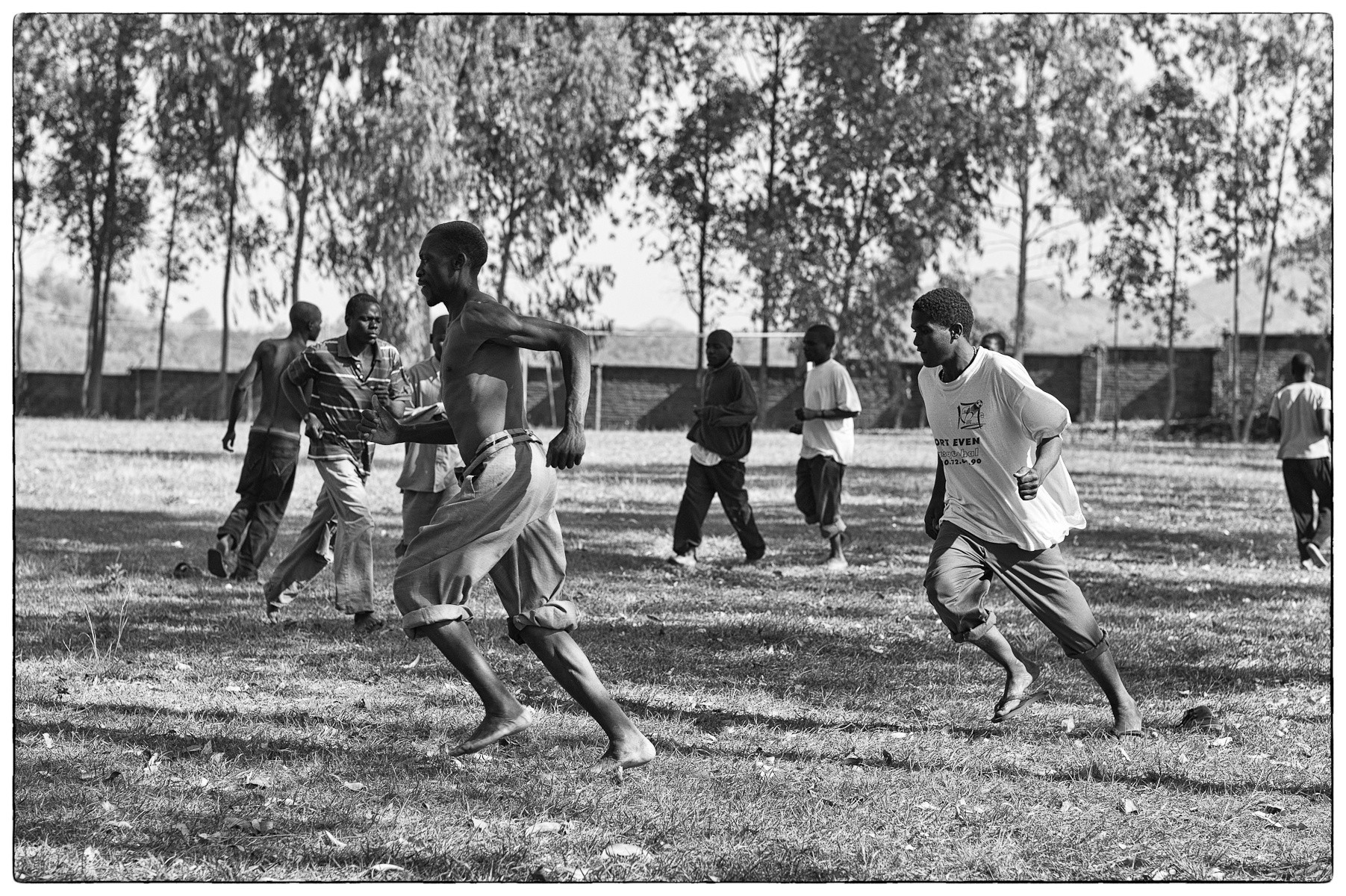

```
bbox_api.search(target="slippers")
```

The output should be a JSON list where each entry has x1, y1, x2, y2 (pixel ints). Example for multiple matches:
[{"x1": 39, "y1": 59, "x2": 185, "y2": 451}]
[
  {"x1": 990, "y1": 690, "x2": 1050, "y2": 724},
  {"x1": 1108, "y1": 727, "x2": 1145, "y2": 748},
  {"x1": 207, "y1": 549, "x2": 227, "y2": 578}
]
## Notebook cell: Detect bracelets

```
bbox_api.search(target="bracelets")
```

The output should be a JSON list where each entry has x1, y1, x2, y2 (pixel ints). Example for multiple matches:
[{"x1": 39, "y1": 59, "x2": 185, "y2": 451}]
[{"x1": 821, "y1": 410, "x2": 825, "y2": 418}]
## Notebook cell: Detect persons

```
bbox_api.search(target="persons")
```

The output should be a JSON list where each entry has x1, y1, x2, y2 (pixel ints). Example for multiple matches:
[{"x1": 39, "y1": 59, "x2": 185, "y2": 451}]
[
  {"x1": 981, "y1": 332, "x2": 1006, "y2": 355},
  {"x1": 1266, "y1": 353, "x2": 1333, "y2": 572},
  {"x1": 396, "y1": 314, "x2": 467, "y2": 546},
  {"x1": 668, "y1": 329, "x2": 769, "y2": 567},
  {"x1": 790, "y1": 324, "x2": 862, "y2": 568},
  {"x1": 262, "y1": 293, "x2": 412, "y2": 633},
  {"x1": 207, "y1": 301, "x2": 323, "y2": 581},
  {"x1": 393, "y1": 221, "x2": 656, "y2": 776},
  {"x1": 911, "y1": 287, "x2": 1144, "y2": 741}
]
